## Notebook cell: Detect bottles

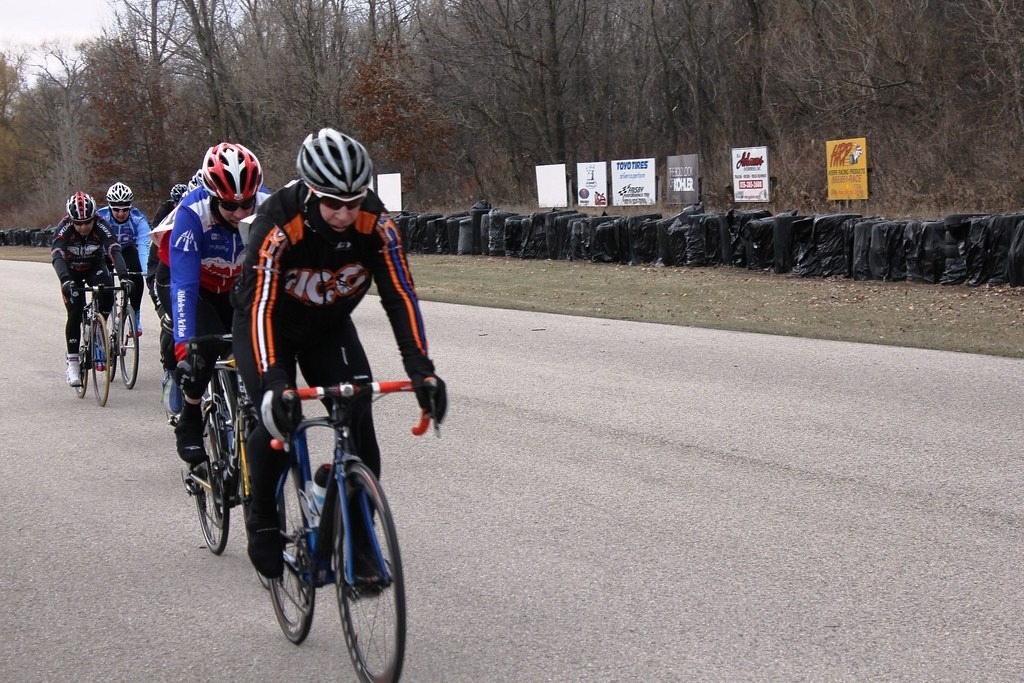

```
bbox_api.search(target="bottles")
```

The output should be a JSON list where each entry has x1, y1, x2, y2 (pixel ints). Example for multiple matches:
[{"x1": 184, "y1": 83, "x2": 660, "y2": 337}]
[
  {"x1": 84, "y1": 318, "x2": 91, "y2": 342},
  {"x1": 115, "y1": 313, "x2": 120, "y2": 330},
  {"x1": 309, "y1": 463, "x2": 333, "y2": 518}
]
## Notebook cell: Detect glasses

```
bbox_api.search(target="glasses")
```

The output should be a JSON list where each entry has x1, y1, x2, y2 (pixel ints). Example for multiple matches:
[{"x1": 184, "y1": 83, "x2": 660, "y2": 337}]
[
  {"x1": 72, "y1": 218, "x2": 94, "y2": 226},
  {"x1": 303, "y1": 181, "x2": 367, "y2": 211},
  {"x1": 109, "y1": 204, "x2": 130, "y2": 212},
  {"x1": 215, "y1": 195, "x2": 256, "y2": 212}
]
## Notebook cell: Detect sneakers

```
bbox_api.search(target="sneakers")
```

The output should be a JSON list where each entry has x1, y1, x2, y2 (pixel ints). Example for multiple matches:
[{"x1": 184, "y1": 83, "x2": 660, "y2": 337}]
[
  {"x1": 174, "y1": 413, "x2": 206, "y2": 463},
  {"x1": 129, "y1": 310, "x2": 142, "y2": 337},
  {"x1": 343, "y1": 507, "x2": 391, "y2": 590},
  {"x1": 96, "y1": 326, "x2": 111, "y2": 353},
  {"x1": 162, "y1": 368, "x2": 182, "y2": 414},
  {"x1": 95, "y1": 346, "x2": 107, "y2": 371},
  {"x1": 247, "y1": 505, "x2": 283, "y2": 579},
  {"x1": 66, "y1": 359, "x2": 82, "y2": 386}
]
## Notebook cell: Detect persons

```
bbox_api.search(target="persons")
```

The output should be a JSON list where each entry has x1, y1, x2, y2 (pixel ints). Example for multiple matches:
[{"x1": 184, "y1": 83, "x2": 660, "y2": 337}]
[
  {"x1": 147, "y1": 169, "x2": 204, "y2": 416},
  {"x1": 51, "y1": 191, "x2": 133, "y2": 387},
  {"x1": 232, "y1": 128, "x2": 447, "y2": 595},
  {"x1": 171, "y1": 142, "x2": 271, "y2": 465},
  {"x1": 96, "y1": 182, "x2": 152, "y2": 370}
]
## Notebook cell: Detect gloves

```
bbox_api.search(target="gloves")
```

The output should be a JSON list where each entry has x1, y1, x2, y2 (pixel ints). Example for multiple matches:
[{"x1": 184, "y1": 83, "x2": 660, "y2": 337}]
[
  {"x1": 158, "y1": 306, "x2": 174, "y2": 336},
  {"x1": 261, "y1": 365, "x2": 302, "y2": 442},
  {"x1": 405, "y1": 358, "x2": 446, "y2": 423},
  {"x1": 61, "y1": 276, "x2": 77, "y2": 305},
  {"x1": 119, "y1": 274, "x2": 136, "y2": 297},
  {"x1": 173, "y1": 352, "x2": 205, "y2": 389}
]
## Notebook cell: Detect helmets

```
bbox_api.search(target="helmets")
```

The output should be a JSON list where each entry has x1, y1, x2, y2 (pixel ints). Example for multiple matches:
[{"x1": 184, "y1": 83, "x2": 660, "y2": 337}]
[
  {"x1": 66, "y1": 191, "x2": 97, "y2": 222},
  {"x1": 181, "y1": 169, "x2": 203, "y2": 199},
  {"x1": 296, "y1": 128, "x2": 373, "y2": 202},
  {"x1": 106, "y1": 182, "x2": 133, "y2": 205},
  {"x1": 201, "y1": 142, "x2": 263, "y2": 203},
  {"x1": 170, "y1": 183, "x2": 188, "y2": 202}
]
